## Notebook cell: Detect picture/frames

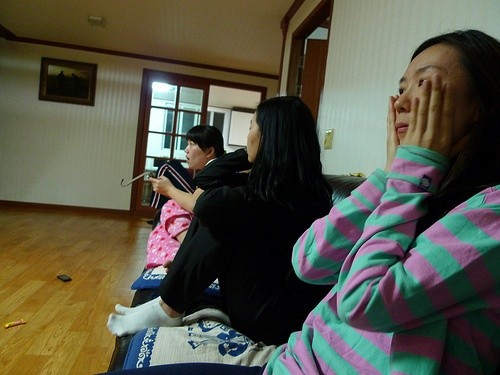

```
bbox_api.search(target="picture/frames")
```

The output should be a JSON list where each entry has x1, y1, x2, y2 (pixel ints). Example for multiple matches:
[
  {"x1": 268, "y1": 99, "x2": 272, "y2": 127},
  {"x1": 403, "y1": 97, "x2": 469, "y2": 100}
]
[{"x1": 38, "y1": 57, "x2": 98, "y2": 107}]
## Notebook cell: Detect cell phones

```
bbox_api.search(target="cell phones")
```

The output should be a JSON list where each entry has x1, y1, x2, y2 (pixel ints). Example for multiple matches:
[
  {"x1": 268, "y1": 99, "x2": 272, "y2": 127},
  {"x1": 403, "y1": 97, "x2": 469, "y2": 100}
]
[{"x1": 57, "y1": 274, "x2": 72, "y2": 281}]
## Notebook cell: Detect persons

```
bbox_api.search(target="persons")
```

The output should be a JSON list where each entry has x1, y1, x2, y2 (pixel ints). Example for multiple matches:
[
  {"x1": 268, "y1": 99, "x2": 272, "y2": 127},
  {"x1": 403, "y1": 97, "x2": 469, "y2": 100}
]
[
  {"x1": 146, "y1": 125, "x2": 256, "y2": 230},
  {"x1": 107, "y1": 95, "x2": 334, "y2": 346},
  {"x1": 89, "y1": 30, "x2": 500, "y2": 375}
]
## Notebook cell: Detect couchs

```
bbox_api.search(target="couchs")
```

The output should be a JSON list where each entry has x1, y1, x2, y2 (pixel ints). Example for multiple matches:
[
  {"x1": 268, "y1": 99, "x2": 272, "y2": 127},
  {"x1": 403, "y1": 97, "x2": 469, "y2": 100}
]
[{"x1": 105, "y1": 173, "x2": 367, "y2": 372}]
